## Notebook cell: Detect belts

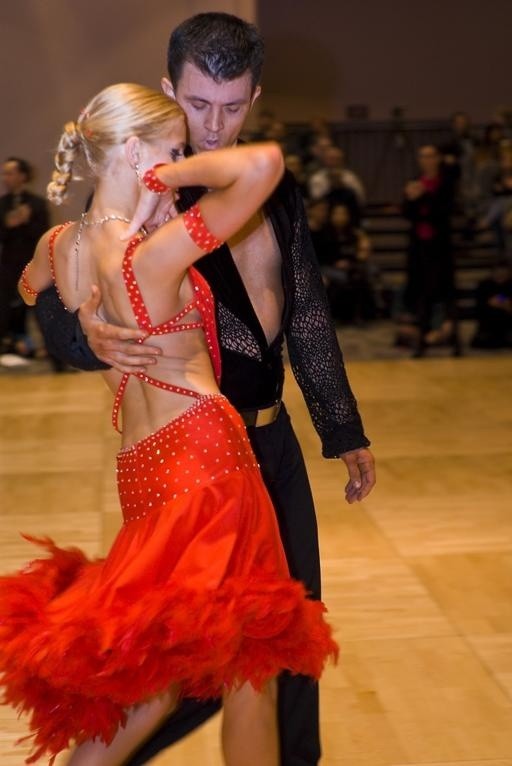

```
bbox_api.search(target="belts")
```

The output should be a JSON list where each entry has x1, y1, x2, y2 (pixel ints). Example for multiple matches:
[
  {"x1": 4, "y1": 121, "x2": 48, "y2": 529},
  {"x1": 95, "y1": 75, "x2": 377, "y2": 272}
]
[{"x1": 241, "y1": 400, "x2": 282, "y2": 427}]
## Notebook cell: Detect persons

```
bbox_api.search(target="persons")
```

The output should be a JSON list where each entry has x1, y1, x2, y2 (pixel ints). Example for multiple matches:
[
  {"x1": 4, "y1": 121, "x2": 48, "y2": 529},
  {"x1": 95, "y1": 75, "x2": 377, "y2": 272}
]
[
  {"x1": 252, "y1": 107, "x2": 512, "y2": 357},
  {"x1": 126, "y1": 13, "x2": 378, "y2": 765},
  {"x1": 1, "y1": 158, "x2": 50, "y2": 366},
  {"x1": 0, "y1": 82, "x2": 341, "y2": 765}
]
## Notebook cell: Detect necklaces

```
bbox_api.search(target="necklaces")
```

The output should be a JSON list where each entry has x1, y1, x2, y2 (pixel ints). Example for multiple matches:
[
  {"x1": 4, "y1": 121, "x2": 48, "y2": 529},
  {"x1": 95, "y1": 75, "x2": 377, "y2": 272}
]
[{"x1": 72, "y1": 213, "x2": 147, "y2": 289}]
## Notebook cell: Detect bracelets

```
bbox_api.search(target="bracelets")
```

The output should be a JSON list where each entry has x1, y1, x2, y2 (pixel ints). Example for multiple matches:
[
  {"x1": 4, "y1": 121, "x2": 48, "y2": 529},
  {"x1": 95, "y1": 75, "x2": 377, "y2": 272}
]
[{"x1": 142, "y1": 162, "x2": 169, "y2": 194}]
